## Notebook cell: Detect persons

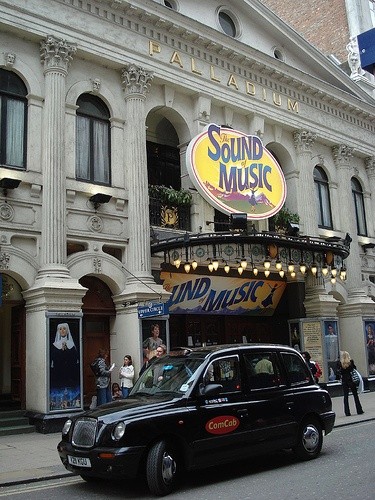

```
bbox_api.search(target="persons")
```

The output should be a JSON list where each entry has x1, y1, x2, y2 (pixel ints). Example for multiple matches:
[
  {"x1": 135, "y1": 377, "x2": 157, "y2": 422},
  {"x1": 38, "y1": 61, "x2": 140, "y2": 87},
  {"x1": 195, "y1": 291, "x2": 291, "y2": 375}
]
[
  {"x1": 366, "y1": 323, "x2": 375, "y2": 374},
  {"x1": 50, "y1": 323, "x2": 77, "y2": 389},
  {"x1": 325, "y1": 324, "x2": 338, "y2": 361},
  {"x1": 96, "y1": 345, "x2": 322, "y2": 406},
  {"x1": 337, "y1": 351, "x2": 364, "y2": 416},
  {"x1": 144, "y1": 324, "x2": 162, "y2": 363}
]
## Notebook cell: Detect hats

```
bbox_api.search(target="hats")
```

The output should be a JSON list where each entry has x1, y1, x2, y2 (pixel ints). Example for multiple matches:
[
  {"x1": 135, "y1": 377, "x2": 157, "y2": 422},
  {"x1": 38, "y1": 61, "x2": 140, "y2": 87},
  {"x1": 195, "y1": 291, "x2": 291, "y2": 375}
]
[{"x1": 159, "y1": 344, "x2": 167, "y2": 350}]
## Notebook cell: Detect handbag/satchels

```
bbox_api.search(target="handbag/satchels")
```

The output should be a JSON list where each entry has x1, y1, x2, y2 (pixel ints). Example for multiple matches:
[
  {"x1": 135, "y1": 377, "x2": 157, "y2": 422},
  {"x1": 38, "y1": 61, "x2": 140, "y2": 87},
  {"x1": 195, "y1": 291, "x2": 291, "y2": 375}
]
[{"x1": 350, "y1": 360, "x2": 360, "y2": 385}]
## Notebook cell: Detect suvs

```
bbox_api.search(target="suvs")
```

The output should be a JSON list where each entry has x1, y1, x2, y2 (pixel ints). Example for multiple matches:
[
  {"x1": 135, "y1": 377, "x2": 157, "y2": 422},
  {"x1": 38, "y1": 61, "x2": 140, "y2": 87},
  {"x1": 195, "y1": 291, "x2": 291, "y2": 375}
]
[{"x1": 56, "y1": 341, "x2": 337, "y2": 497}]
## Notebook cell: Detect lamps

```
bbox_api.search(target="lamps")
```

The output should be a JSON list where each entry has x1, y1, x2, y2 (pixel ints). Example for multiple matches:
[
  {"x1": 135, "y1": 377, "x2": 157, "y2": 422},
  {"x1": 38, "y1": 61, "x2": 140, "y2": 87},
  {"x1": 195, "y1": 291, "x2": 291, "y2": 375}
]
[
  {"x1": 285, "y1": 222, "x2": 300, "y2": 236},
  {"x1": 89, "y1": 193, "x2": 111, "y2": 209},
  {"x1": 326, "y1": 234, "x2": 342, "y2": 242},
  {"x1": 362, "y1": 241, "x2": 375, "y2": 248},
  {"x1": 0, "y1": 178, "x2": 22, "y2": 196},
  {"x1": 229, "y1": 213, "x2": 248, "y2": 232},
  {"x1": 173, "y1": 244, "x2": 347, "y2": 284}
]
